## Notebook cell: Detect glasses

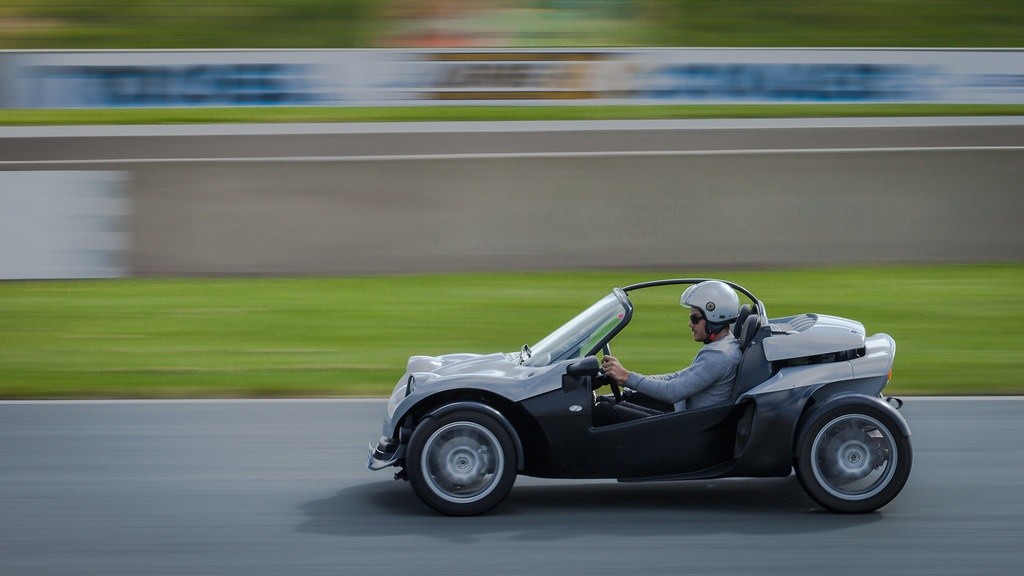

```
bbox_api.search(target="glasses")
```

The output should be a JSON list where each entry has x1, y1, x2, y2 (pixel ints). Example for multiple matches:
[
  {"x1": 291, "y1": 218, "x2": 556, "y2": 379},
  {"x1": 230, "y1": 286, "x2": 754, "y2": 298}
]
[{"x1": 690, "y1": 314, "x2": 703, "y2": 325}]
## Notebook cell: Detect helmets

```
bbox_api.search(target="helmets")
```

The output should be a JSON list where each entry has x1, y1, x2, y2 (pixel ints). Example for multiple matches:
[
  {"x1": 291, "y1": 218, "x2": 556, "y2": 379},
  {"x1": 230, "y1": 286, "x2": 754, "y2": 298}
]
[{"x1": 680, "y1": 281, "x2": 741, "y2": 344}]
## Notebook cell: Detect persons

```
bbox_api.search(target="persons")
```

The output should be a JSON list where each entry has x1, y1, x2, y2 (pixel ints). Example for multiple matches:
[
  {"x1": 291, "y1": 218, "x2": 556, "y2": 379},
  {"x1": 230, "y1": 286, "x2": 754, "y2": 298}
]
[{"x1": 591, "y1": 280, "x2": 742, "y2": 428}]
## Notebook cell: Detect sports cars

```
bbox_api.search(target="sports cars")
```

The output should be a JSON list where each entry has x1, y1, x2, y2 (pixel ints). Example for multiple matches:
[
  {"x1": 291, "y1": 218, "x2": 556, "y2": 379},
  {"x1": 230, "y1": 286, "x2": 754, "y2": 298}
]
[{"x1": 366, "y1": 276, "x2": 916, "y2": 522}]
